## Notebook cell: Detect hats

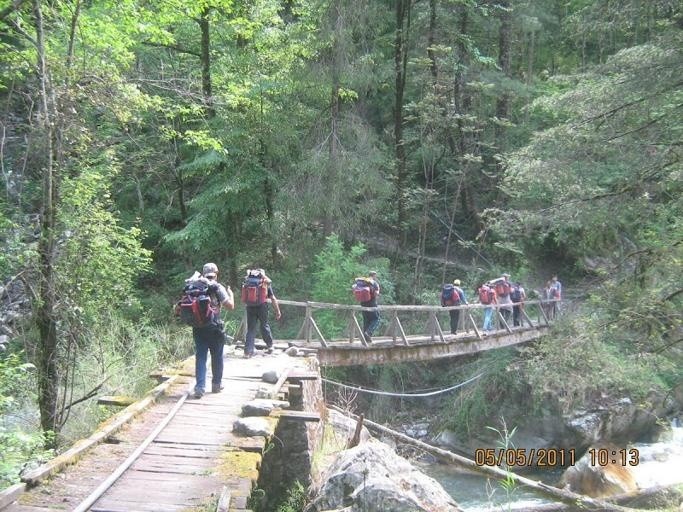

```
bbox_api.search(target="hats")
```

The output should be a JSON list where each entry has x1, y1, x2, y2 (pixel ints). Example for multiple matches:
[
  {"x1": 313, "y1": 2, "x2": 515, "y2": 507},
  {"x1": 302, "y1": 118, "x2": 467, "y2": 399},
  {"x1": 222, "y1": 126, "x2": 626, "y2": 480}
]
[{"x1": 203, "y1": 263, "x2": 218, "y2": 277}]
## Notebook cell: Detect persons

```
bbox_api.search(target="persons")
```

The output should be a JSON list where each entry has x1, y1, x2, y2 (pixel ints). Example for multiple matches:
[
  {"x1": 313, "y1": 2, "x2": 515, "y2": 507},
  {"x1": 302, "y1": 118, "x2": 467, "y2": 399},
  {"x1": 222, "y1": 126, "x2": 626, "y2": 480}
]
[
  {"x1": 450, "y1": 279, "x2": 469, "y2": 335},
  {"x1": 243, "y1": 269, "x2": 281, "y2": 358},
  {"x1": 482, "y1": 273, "x2": 561, "y2": 330},
  {"x1": 193, "y1": 263, "x2": 234, "y2": 399},
  {"x1": 360, "y1": 271, "x2": 380, "y2": 345}
]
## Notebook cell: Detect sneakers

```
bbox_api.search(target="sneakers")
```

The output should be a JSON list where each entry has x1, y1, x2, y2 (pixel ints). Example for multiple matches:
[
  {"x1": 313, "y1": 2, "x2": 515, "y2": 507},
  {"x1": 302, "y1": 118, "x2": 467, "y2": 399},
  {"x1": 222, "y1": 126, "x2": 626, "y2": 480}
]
[
  {"x1": 213, "y1": 386, "x2": 223, "y2": 392},
  {"x1": 363, "y1": 332, "x2": 372, "y2": 343}
]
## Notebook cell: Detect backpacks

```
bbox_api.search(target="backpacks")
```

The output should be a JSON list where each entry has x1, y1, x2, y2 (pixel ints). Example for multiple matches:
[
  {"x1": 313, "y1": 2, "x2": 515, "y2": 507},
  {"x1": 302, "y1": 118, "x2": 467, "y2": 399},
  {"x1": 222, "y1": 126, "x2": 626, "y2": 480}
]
[
  {"x1": 241, "y1": 270, "x2": 271, "y2": 307},
  {"x1": 511, "y1": 287, "x2": 522, "y2": 302},
  {"x1": 175, "y1": 280, "x2": 220, "y2": 328},
  {"x1": 478, "y1": 285, "x2": 495, "y2": 304},
  {"x1": 441, "y1": 284, "x2": 460, "y2": 305},
  {"x1": 496, "y1": 280, "x2": 510, "y2": 297},
  {"x1": 352, "y1": 278, "x2": 372, "y2": 302}
]
